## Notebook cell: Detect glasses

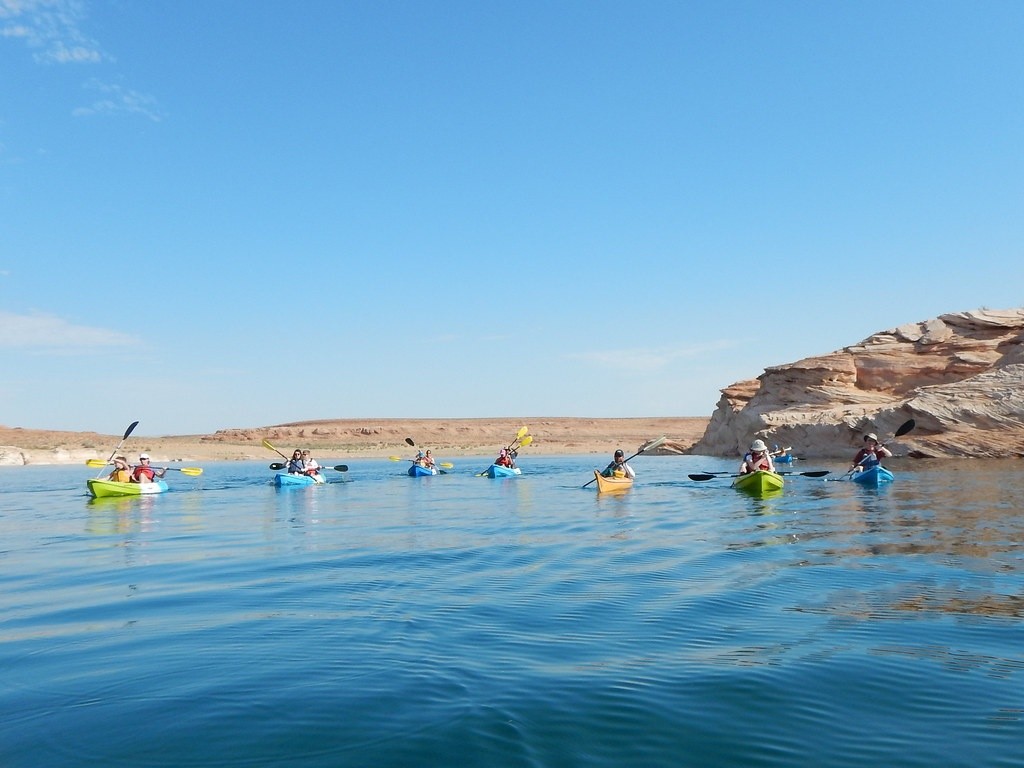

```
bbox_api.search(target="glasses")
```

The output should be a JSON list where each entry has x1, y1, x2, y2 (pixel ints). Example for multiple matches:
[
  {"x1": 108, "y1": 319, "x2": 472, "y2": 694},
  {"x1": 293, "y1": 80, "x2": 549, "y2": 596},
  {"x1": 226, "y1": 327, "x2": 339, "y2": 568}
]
[
  {"x1": 295, "y1": 454, "x2": 300, "y2": 455},
  {"x1": 140, "y1": 458, "x2": 147, "y2": 460}
]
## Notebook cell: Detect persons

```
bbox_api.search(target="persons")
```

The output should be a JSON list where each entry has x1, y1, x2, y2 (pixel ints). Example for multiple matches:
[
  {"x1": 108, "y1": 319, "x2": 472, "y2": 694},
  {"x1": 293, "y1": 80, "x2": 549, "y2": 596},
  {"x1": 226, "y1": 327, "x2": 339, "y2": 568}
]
[
  {"x1": 849, "y1": 433, "x2": 892, "y2": 473},
  {"x1": 601, "y1": 449, "x2": 635, "y2": 479},
  {"x1": 130, "y1": 454, "x2": 167, "y2": 484},
  {"x1": 739, "y1": 439, "x2": 786, "y2": 476},
  {"x1": 95, "y1": 456, "x2": 130, "y2": 483},
  {"x1": 495, "y1": 446, "x2": 519, "y2": 469},
  {"x1": 412, "y1": 450, "x2": 437, "y2": 469},
  {"x1": 286, "y1": 449, "x2": 321, "y2": 477}
]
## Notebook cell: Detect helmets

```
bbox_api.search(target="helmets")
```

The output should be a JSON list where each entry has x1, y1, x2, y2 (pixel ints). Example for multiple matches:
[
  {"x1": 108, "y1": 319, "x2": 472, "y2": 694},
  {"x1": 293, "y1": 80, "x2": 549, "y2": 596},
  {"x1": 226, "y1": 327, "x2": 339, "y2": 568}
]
[{"x1": 772, "y1": 445, "x2": 778, "y2": 449}]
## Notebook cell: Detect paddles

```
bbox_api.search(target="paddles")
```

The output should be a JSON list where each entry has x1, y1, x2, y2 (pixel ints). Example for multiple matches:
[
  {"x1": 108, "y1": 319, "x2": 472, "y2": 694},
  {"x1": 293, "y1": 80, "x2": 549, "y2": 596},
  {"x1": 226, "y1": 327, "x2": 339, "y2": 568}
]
[
  {"x1": 509, "y1": 426, "x2": 528, "y2": 448},
  {"x1": 269, "y1": 463, "x2": 348, "y2": 472},
  {"x1": 583, "y1": 436, "x2": 666, "y2": 487},
  {"x1": 85, "y1": 459, "x2": 203, "y2": 476},
  {"x1": 481, "y1": 436, "x2": 532, "y2": 476},
  {"x1": 86, "y1": 421, "x2": 139, "y2": 493},
  {"x1": 389, "y1": 456, "x2": 453, "y2": 469},
  {"x1": 769, "y1": 446, "x2": 792, "y2": 455},
  {"x1": 405, "y1": 438, "x2": 446, "y2": 474},
  {"x1": 262, "y1": 439, "x2": 320, "y2": 484},
  {"x1": 839, "y1": 419, "x2": 916, "y2": 479},
  {"x1": 688, "y1": 470, "x2": 832, "y2": 481},
  {"x1": 793, "y1": 457, "x2": 806, "y2": 460}
]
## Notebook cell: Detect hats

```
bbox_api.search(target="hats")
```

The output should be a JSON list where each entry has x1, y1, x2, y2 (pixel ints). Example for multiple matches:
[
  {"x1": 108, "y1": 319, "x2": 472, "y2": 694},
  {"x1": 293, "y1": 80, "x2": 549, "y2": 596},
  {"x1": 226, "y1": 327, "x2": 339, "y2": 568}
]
[
  {"x1": 615, "y1": 450, "x2": 624, "y2": 457},
  {"x1": 419, "y1": 453, "x2": 425, "y2": 457},
  {"x1": 115, "y1": 454, "x2": 126, "y2": 462},
  {"x1": 864, "y1": 433, "x2": 879, "y2": 446},
  {"x1": 504, "y1": 446, "x2": 510, "y2": 450},
  {"x1": 750, "y1": 439, "x2": 767, "y2": 451},
  {"x1": 139, "y1": 454, "x2": 149, "y2": 458},
  {"x1": 500, "y1": 450, "x2": 506, "y2": 454}
]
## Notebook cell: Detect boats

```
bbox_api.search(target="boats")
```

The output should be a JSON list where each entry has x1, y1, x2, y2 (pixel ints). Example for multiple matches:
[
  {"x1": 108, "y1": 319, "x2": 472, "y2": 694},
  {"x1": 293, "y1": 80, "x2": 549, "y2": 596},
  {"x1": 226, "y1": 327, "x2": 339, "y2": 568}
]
[
  {"x1": 594, "y1": 469, "x2": 634, "y2": 492},
  {"x1": 86, "y1": 478, "x2": 168, "y2": 498},
  {"x1": 733, "y1": 470, "x2": 784, "y2": 492},
  {"x1": 488, "y1": 464, "x2": 517, "y2": 478},
  {"x1": 408, "y1": 464, "x2": 432, "y2": 477},
  {"x1": 275, "y1": 473, "x2": 315, "y2": 485},
  {"x1": 849, "y1": 464, "x2": 894, "y2": 483}
]
